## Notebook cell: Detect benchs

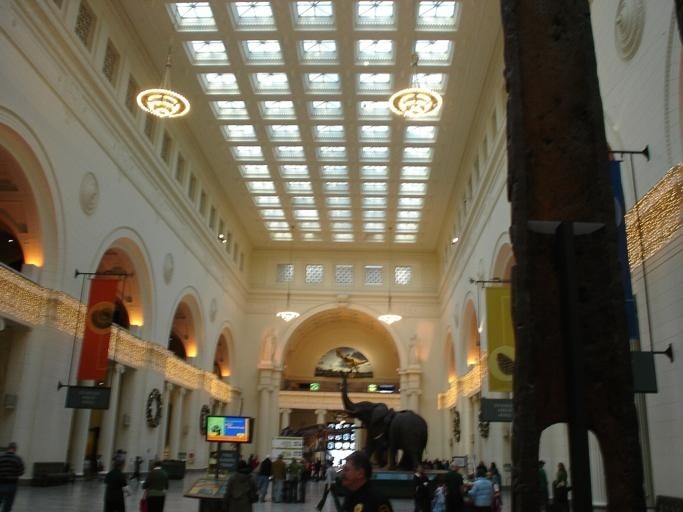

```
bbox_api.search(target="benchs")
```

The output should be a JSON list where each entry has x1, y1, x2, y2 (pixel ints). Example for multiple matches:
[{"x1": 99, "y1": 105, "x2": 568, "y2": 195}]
[{"x1": 33, "y1": 463, "x2": 72, "y2": 487}]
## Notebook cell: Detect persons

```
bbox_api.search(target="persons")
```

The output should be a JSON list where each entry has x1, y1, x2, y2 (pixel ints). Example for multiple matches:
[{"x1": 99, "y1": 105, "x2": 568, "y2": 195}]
[
  {"x1": 539, "y1": 461, "x2": 568, "y2": 506},
  {"x1": 90, "y1": 449, "x2": 168, "y2": 511},
  {"x1": 412, "y1": 458, "x2": 501, "y2": 510},
  {"x1": 222, "y1": 451, "x2": 394, "y2": 511},
  {"x1": 0, "y1": 443, "x2": 25, "y2": 512}
]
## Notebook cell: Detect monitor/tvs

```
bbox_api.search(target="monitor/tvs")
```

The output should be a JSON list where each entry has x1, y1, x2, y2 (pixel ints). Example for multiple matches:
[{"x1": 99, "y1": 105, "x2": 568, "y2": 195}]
[{"x1": 205, "y1": 414, "x2": 254, "y2": 443}]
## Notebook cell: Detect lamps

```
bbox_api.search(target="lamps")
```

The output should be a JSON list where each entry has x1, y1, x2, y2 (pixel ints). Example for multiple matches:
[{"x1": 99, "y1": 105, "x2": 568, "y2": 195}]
[
  {"x1": 378, "y1": 226, "x2": 402, "y2": 324},
  {"x1": 388, "y1": 0, "x2": 443, "y2": 117},
  {"x1": 277, "y1": 226, "x2": 300, "y2": 322},
  {"x1": 136, "y1": 46, "x2": 191, "y2": 118}
]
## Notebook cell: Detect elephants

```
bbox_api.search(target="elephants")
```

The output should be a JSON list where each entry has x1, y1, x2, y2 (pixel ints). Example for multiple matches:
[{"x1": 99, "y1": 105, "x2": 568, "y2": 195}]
[{"x1": 339, "y1": 370, "x2": 428, "y2": 471}]
